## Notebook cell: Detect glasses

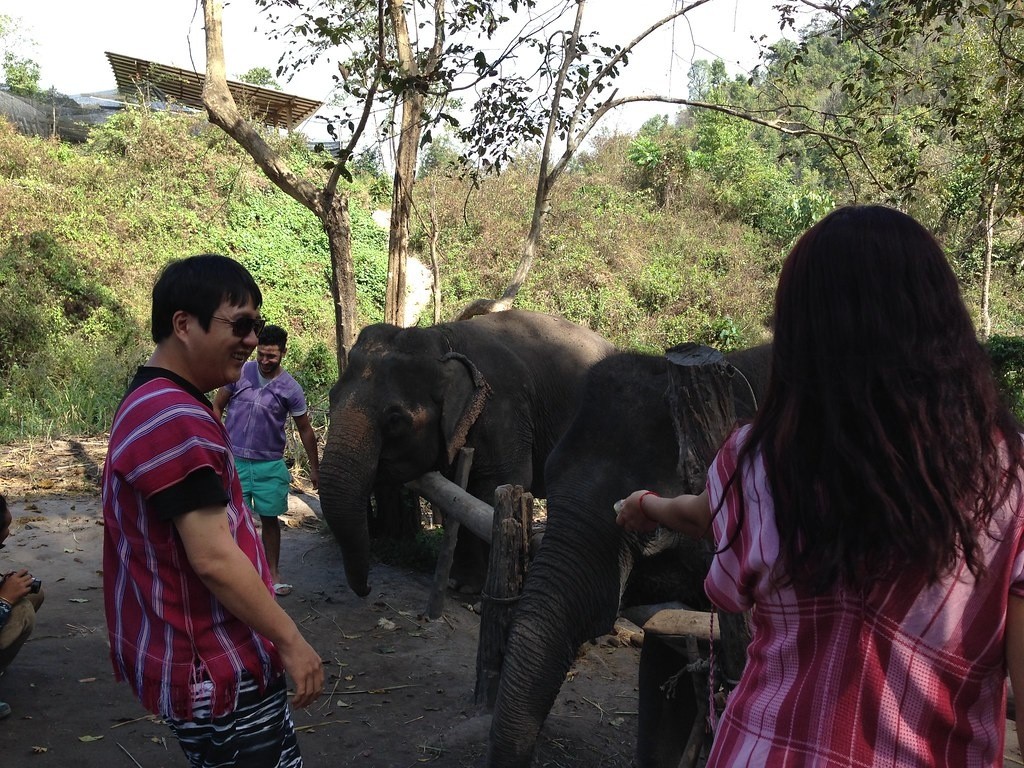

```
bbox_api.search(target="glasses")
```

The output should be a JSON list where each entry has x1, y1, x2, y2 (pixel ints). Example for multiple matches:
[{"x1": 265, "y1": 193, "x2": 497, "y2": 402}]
[{"x1": 180, "y1": 309, "x2": 267, "y2": 338}]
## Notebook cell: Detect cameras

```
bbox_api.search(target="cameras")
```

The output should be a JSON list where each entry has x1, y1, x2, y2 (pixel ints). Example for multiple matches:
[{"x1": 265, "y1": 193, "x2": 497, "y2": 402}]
[{"x1": 10, "y1": 571, "x2": 41, "y2": 594}]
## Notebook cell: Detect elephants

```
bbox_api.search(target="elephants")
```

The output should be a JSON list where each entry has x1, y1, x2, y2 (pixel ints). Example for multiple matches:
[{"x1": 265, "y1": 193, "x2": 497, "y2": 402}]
[{"x1": 313, "y1": 307, "x2": 779, "y2": 768}]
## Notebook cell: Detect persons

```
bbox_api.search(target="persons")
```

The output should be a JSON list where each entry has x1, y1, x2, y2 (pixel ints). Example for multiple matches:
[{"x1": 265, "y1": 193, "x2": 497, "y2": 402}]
[
  {"x1": 613, "y1": 205, "x2": 1024, "y2": 766},
  {"x1": 213, "y1": 325, "x2": 321, "y2": 595},
  {"x1": 100, "y1": 255, "x2": 324, "y2": 768},
  {"x1": 0, "y1": 495, "x2": 45, "y2": 717}
]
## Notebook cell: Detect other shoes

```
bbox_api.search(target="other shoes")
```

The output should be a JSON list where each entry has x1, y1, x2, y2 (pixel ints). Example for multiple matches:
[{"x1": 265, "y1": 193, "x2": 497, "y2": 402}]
[{"x1": 0, "y1": 702, "x2": 11, "y2": 718}]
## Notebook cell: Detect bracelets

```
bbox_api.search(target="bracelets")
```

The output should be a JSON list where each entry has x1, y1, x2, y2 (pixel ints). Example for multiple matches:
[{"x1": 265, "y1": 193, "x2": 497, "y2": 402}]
[
  {"x1": 638, "y1": 491, "x2": 661, "y2": 520},
  {"x1": 0, "y1": 596, "x2": 14, "y2": 605}
]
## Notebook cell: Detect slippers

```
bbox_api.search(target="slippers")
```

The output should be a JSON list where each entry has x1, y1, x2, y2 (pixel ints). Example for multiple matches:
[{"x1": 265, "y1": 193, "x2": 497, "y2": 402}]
[{"x1": 271, "y1": 583, "x2": 292, "y2": 595}]
